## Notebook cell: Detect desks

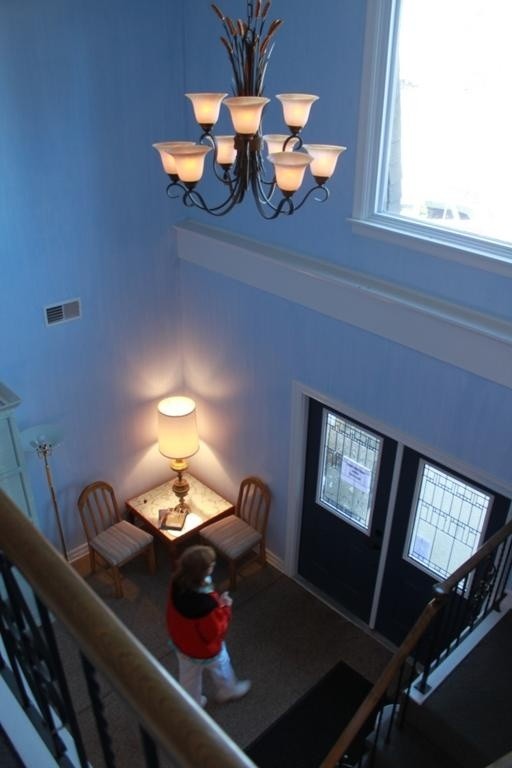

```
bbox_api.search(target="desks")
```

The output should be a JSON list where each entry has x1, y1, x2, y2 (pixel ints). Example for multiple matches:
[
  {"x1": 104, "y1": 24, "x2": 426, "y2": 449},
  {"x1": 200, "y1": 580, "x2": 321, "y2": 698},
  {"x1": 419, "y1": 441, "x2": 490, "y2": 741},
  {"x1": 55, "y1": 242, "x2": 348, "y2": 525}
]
[{"x1": 124, "y1": 470, "x2": 236, "y2": 576}]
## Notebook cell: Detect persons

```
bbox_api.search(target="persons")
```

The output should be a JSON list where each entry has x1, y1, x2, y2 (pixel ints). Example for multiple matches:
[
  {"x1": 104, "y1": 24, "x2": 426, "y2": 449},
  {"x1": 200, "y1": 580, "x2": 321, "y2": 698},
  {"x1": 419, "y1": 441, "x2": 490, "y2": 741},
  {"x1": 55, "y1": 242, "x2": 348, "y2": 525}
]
[{"x1": 166, "y1": 544, "x2": 252, "y2": 709}]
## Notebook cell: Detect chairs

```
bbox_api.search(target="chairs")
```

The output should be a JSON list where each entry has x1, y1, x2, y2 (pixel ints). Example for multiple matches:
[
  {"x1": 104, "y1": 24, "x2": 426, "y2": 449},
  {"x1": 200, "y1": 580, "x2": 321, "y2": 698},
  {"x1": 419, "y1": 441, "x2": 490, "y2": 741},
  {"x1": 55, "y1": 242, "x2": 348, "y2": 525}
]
[
  {"x1": 77, "y1": 480, "x2": 156, "y2": 600},
  {"x1": 198, "y1": 476, "x2": 271, "y2": 593}
]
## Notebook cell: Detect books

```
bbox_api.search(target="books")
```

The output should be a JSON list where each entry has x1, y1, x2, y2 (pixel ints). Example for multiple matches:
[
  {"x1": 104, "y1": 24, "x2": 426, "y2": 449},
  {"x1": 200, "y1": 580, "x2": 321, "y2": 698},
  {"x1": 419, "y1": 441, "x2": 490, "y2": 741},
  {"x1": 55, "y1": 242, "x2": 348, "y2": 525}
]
[{"x1": 157, "y1": 506, "x2": 188, "y2": 531}]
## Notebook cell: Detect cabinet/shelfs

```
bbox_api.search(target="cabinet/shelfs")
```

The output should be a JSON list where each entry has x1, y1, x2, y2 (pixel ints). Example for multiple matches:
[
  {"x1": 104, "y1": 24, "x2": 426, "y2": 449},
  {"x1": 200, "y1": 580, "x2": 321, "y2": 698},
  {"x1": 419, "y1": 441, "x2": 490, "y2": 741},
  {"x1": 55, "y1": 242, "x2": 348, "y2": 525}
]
[{"x1": 1, "y1": 382, "x2": 57, "y2": 632}]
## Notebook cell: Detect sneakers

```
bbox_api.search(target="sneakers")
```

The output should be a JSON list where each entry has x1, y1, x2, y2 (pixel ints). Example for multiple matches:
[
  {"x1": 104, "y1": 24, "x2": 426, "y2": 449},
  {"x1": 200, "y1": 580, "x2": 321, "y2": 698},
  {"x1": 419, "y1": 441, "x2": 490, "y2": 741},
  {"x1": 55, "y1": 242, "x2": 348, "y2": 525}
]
[
  {"x1": 200, "y1": 696, "x2": 207, "y2": 708},
  {"x1": 217, "y1": 679, "x2": 251, "y2": 702}
]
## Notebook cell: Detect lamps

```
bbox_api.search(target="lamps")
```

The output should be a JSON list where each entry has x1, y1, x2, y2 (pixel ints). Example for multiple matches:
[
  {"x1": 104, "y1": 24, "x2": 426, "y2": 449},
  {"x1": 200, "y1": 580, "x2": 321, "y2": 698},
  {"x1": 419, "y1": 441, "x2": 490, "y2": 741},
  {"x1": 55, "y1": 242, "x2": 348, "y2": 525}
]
[
  {"x1": 151, "y1": 1, "x2": 347, "y2": 220},
  {"x1": 21, "y1": 423, "x2": 69, "y2": 563},
  {"x1": 156, "y1": 396, "x2": 201, "y2": 511}
]
